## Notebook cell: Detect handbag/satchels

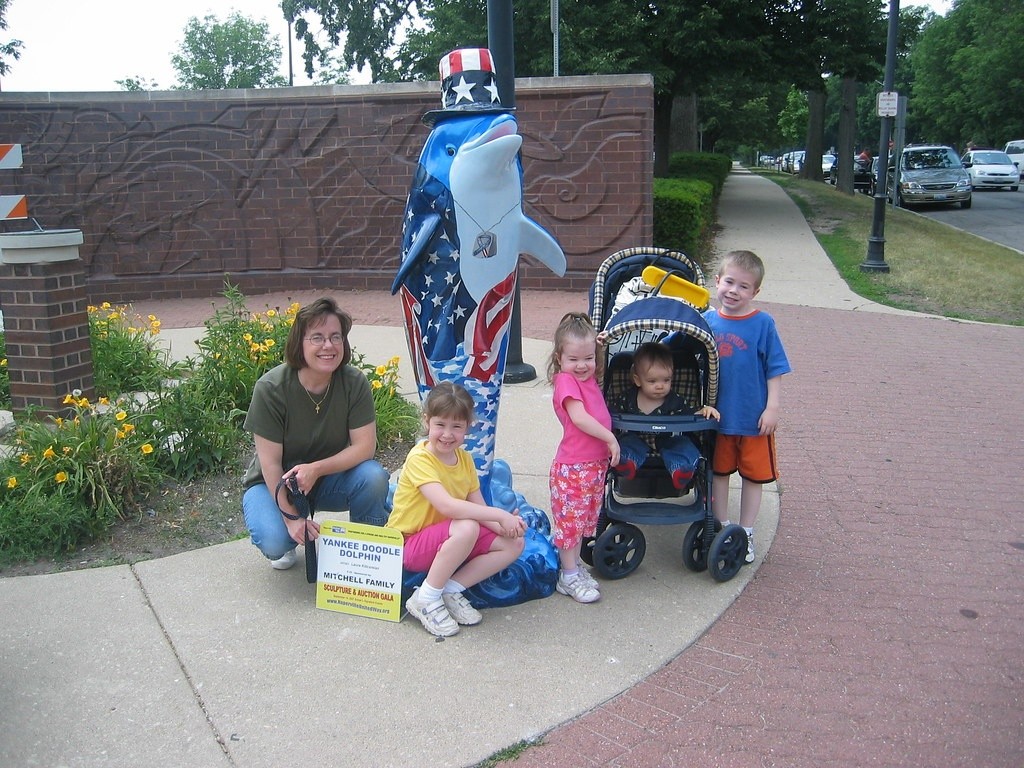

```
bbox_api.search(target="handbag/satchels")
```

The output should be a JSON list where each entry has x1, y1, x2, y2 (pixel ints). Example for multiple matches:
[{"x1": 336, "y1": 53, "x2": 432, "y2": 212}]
[{"x1": 603, "y1": 248, "x2": 717, "y2": 364}]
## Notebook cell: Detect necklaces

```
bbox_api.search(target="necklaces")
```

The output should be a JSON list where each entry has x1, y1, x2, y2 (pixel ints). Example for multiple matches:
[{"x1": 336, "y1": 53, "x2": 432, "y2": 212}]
[{"x1": 299, "y1": 370, "x2": 331, "y2": 413}]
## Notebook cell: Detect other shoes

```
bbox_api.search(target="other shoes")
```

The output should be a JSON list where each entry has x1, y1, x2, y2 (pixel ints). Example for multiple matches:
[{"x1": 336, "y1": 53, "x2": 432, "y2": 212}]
[{"x1": 271, "y1": 548, "x2": 296, "y2": 570}]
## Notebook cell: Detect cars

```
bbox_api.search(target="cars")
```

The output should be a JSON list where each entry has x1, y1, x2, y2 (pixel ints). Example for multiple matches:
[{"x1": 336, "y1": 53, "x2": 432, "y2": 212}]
[
  {"x1": 886, "y1": 143, "x2": 973, "y2": 209},
  {"x1": 959, "y1": 148, "x2": 1020, "y2": 191},
  {"x1": 871, "y1": 154, "x2": 895, "y2": 197},
  {"x1": 759, "y1": 150, "x2": 877, "y2": 197},
  {"x1": 1001, "y1": 141, "x2": 1024, "y2": 180}
]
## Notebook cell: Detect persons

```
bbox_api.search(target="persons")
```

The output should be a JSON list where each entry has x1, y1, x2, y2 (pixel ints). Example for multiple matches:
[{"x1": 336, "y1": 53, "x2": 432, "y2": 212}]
[
  {"x1": 860, "y1": 148, "x2": 873, "y2": 164},
  {"x1": 385, "y1": 381, "x2": 527, "y2": 636},
  {"x1": 611, "y1": 342, "x2": 720, "y2": 489},
  {"x1": 660, "y1": 250, "x2": 790, "y2": 563},
  {"x1": 244, "y1": 298, "x2": 391, "y2": 569},
  {"x1": 547, "y1": 312, "x2": 620, "y2": 603}
]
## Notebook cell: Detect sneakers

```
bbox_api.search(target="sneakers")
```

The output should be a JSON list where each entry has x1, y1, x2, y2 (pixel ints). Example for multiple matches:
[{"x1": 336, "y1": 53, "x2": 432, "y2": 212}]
[
  {"x1": 440, "y1": 592, "x2": 482, "y2": 625},
  {"x1": 721, "y1": 525, "x2": 732, "y2": 544},
  {"x1": 745, "y1": 532, "x2": 754, "y2": 563},
  {"x1": 556, "y1": 572, "x2": 600, "y2": 603},
  {"x1": 405, "y1": 586, "x2": 459, "y2": 637},
  {"x1": 578, "y1": 567, "x2": 599, "y2": 590}
]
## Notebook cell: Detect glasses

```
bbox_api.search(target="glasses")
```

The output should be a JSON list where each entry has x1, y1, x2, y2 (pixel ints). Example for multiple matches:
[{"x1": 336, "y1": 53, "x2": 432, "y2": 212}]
[{"x1": 302, "y1": 334, "x2": 344, "y2": 346}]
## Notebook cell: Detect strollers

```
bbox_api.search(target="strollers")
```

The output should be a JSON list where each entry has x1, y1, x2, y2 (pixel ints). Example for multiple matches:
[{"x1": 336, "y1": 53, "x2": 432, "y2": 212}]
[{"x1": 579, "y1": 246, "x2": 750, "y2": 582}]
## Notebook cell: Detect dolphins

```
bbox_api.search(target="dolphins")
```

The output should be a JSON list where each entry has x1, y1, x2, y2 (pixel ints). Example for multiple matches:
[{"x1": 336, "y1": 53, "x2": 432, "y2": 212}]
[{"x1": 391, "y1": 112, "x2": 567, "y2": 508}]
